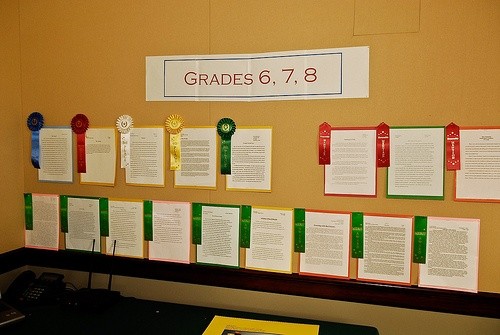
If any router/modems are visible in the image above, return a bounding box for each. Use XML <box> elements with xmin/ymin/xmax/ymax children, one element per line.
<box><xmin>60</xmin><ymin>239</ymin><xmax>123</xmax><ymax>315</ymax></box>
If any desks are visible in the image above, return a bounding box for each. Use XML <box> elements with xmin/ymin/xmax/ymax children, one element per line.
<box><xmin>1</xmin><ymin>299</ymin><xmax>378</xmax><ymax>335</ymax></box>
